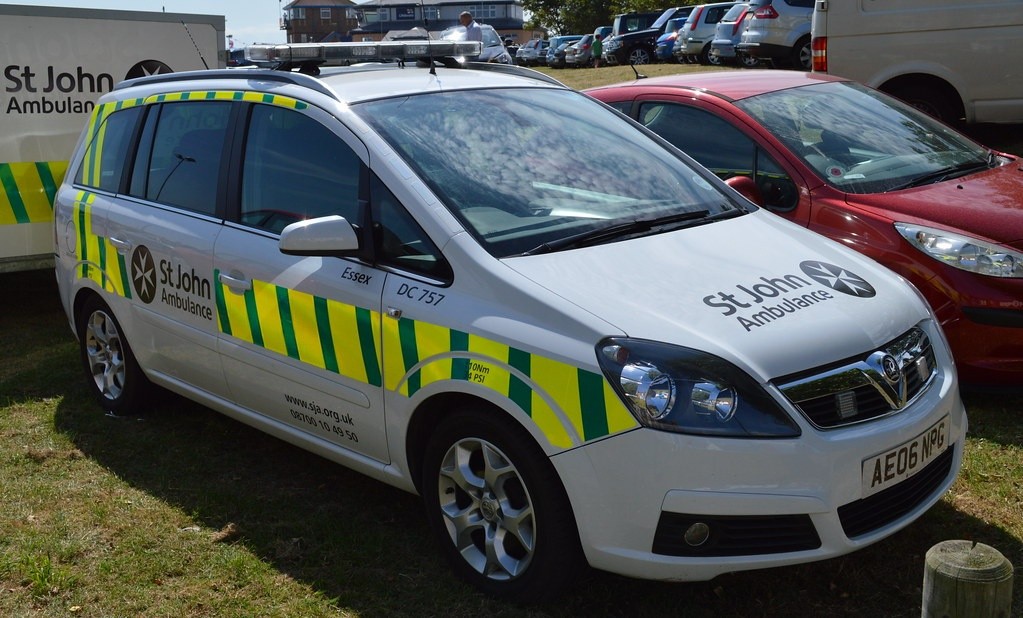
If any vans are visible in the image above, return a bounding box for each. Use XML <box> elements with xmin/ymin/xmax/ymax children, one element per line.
<box><xmin>811</xmin><ymin>0</ymin><xmax>1022</xmax><ymax>130</ymax></box>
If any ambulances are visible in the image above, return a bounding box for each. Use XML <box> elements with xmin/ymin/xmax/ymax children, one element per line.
<box><xmin>52</xmin><ymin>62</ymin><xmax>970</xmax><ymax>602</ymax></box>
<box><xmin>0</xmin><ymin>4</ymin><xmax>225</xmax><ymax>272</ymax></box>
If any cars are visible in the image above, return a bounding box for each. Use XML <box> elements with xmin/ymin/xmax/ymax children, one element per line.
<box><xmin>708</xmin><ymin>4</ymin><xmax>770</xmax><ymax>69</ymax></box>
<box><xmin>526</xmin><ymin>69</ymin><xmax>1023</xmax><ymax>388</ymax></box>
<box><xmin>656</xmin><ymin>18</ymin><xmax>699</xmax><ymax>64</ymax></box>
<box><xmin>439</xmin><ymin>23</ymin><xmax>513</xmax><ymax>65</ymax></box>
<box><xmin>680</xmin><ymin>2</ymin><xmax>749</xmax><ymax>66</ymax></box>
<box><xmin>563</xmin><ymin>9</ymin><xmax>686</xmax><ymax>66</ymax></box>
<box><xmin>515</xmin><ymin>35</ymin><xmax>583</xmax><ymax>69</ymax></box>
<box><xmin>605</xmin><ymin>4</ymin><xmax>728</xmax><ymax>65</ymax></box>
<box><xmin>734</xmin><ymin>0</ymin><xmax>815</xmax><ymax>70</ymax></box>
<box><xmin>240</xmin><ymin>209</ymin><xmax>314</xmax><ymax>235</ymax></box>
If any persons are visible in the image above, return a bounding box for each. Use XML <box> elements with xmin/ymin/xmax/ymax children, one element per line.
<box><xmin>459</xmin><ymin>11</ymin><xmax>482</xmax><ymax>61</ymax></box>
<box><xmin>589</xmin><ymin>35</ymin><xmax>604</xmax><ymax>68</ymax></box>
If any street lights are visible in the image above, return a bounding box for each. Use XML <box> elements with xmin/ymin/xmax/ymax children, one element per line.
<box><xmin>155</xmin><ymin>153</ymin><xmax>197</xmax><ymax>202</ymax></box>
